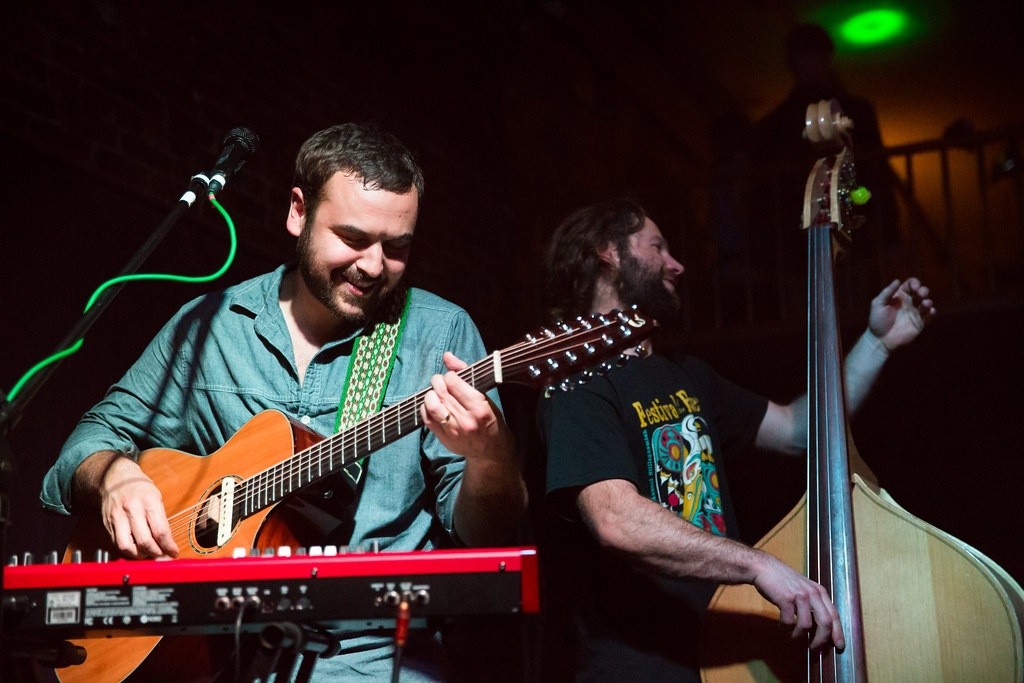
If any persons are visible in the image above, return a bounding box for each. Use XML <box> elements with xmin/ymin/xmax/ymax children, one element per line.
<box><xmin>527</xmin><ymin>196</ymin><xmax>935</xmax><ymax>683</ymax></box>
<box><xmin>39</xmin><ymin>124</ymin><xmax>529</xmax><ymax>682</ymax></box>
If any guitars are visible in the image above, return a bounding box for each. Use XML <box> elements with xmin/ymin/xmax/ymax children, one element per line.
<box><xmin>49</xmin><ymin>298</ymin><xmax>668</xmax><ymax>682</ymax></box>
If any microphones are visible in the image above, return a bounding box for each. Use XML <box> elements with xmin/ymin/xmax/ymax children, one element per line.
<box><xmin>207</xmin><ymin>124</ymin><xmax>261</xmax><ymax>194</ymax></box>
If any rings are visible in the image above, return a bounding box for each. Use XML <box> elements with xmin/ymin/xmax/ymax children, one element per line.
<box><xmin>440</xmin><ymin>414</ymin><xmax>450</xmax><ymax>426</ymax></box>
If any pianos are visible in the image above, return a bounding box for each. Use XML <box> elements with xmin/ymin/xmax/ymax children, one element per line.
<box><xmin>1</xmin><ymin>539</ymin><xmax>542</xmax><ymax>683</ymax></box>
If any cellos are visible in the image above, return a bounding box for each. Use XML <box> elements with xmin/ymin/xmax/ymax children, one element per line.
<box><xmin>696</xmin><ymin>94</ymin><xmax>1024</xmax><ymax>683</ymax></box>
